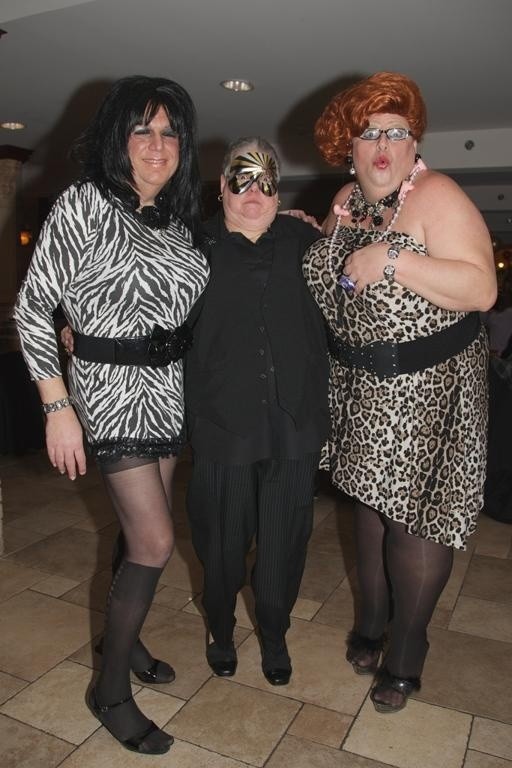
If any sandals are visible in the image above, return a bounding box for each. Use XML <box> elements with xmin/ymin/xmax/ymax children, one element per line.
<box><xmin>369</xmin><ymin>638</ymin><xmax>431</xmax><ymax>713</ymax></box>
<box><xmin>93</xmin><ymin>632</ymin><xmax>176</xmax><ymax>685</ymax></box>
<box><xmin>88</xmin><ymin>682</ymin><xmax>171</xmax><ymax>756</ymax></box>
<box><xmin>345</xmin><ymin>625</ymin><xmax>386</xmax><ymax>675</ymax></box>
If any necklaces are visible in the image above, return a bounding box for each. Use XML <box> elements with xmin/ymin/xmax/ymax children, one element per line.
<box><xmin>327</xmin><ymin>156</ymin><xmax>428</xmax><ymax>285</ymax></box>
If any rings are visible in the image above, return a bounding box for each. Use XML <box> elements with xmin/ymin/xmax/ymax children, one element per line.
<box><xmin>339</xmin><ymin>275</ymin><xmax>355</xmax><ymax>290</ymax></box>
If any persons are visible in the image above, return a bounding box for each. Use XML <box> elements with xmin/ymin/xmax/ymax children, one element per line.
<box><xmin>13</xmin><ymin>76</ymin><xmax>322</xmax><ymax>755</ymax></box>
<box><xmin>301</xmin><ymin>71</ymin><xmax>499</xmax><ymax>713</ymax></box>
<box><xmin>61</xmin><ymin>134</ymin><xmax>331</xmax><ymax>687</ymax></box>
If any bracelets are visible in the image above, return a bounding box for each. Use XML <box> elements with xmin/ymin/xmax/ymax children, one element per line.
<box><xmin>42</xmin><ymin>397</ymin><xmax>71</xmax><ymax>415</ymax></box>
<box><xmin>384</xmin><ymin>245</ymin><xmax>401</xmax><ymax>282</ymax></box>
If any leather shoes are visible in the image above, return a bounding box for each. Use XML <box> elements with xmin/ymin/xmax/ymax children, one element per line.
<box><xmin>259</xmin><ymin>629</ymin><xmax>293</xmax><ymax>686</ymax></box>
<box><xmin>205</xmin><ymin>626</ymin><xmax>238</xmax><ymax>678</ymax></box>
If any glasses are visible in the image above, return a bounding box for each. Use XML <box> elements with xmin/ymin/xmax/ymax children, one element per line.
<box><xmin>359</xmin><ymin>127</ymin><xmax>413</xmax><ymax>142</ymax></box>
